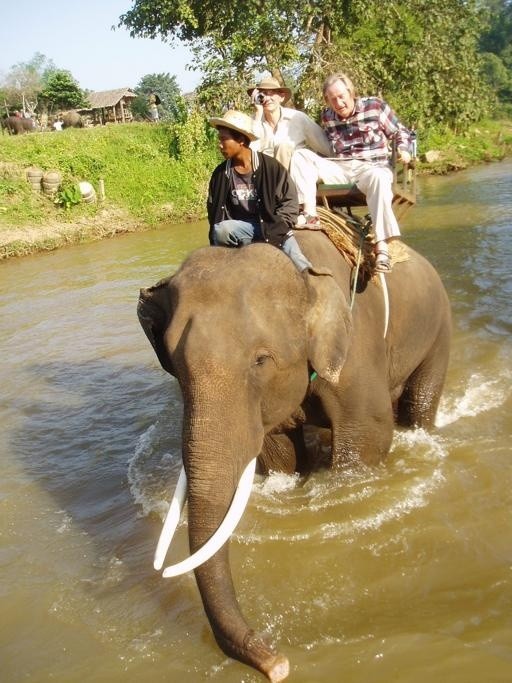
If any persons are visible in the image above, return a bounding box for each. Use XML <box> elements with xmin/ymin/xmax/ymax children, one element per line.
<box><xmin>206</xmin><ymin>75</ymin><xmax>333</xmax><ymax>275</ymax></box>
<box><xmin>146</xmin><ymin>89</ymin><xmax>159</xmax><ymax>122</ymax></box>
<box><xmin>53</xmin><ymin>119</ymin><xmax>64</xmax><ymax>131</ymax></box>
<box><xmin>289</xmin><ymin>72</ymin><xmax>410</xmax><ymax>273</ymax></box>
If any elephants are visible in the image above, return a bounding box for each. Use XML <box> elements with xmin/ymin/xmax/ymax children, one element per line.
<box><xmin>58</xmin><ymin>111</ymin><xmax>84</xmax><ymax>130</ymax></box>
<box><xmin>137</xmin><ymin>204</ymin><xmax>453</xmax><ymax>683</ymax></box>
<box><xmin>0</xmin><ymin>116</ymin><xmax>36</xmax><ymax>136</ymax></box>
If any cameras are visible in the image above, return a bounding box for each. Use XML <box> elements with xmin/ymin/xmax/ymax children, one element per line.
<box><xmin>253</xmin><ymin>92</ymin><xmax>268</xmax><ymax>106</ymax></box>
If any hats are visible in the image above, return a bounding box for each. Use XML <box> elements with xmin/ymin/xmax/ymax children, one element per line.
<box><xmin>209</xmin><ymin>110</ymin><xmax>262</xmax><ymax>142</ymax></box>
<box><xmin>245</xmin><ymin>76</ymin><xmax>292</xmax><ymax>105</ymax></box>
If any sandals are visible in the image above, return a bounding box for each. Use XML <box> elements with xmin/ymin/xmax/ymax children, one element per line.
<box><xmin>373</xmin><ymin>244</ymin><xmax>392</xmax><ymax>273</ymax></box>
<box><xmin>292</xmin><ymin>210</ymin><xmax>321</xmax><ymax>230</ymax></box>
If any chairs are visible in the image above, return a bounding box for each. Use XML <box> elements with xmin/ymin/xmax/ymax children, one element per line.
<box><xmin>318</xmin><ymin>130</ymin><xmax>416</xmax><ymax>227</ymax></box>
